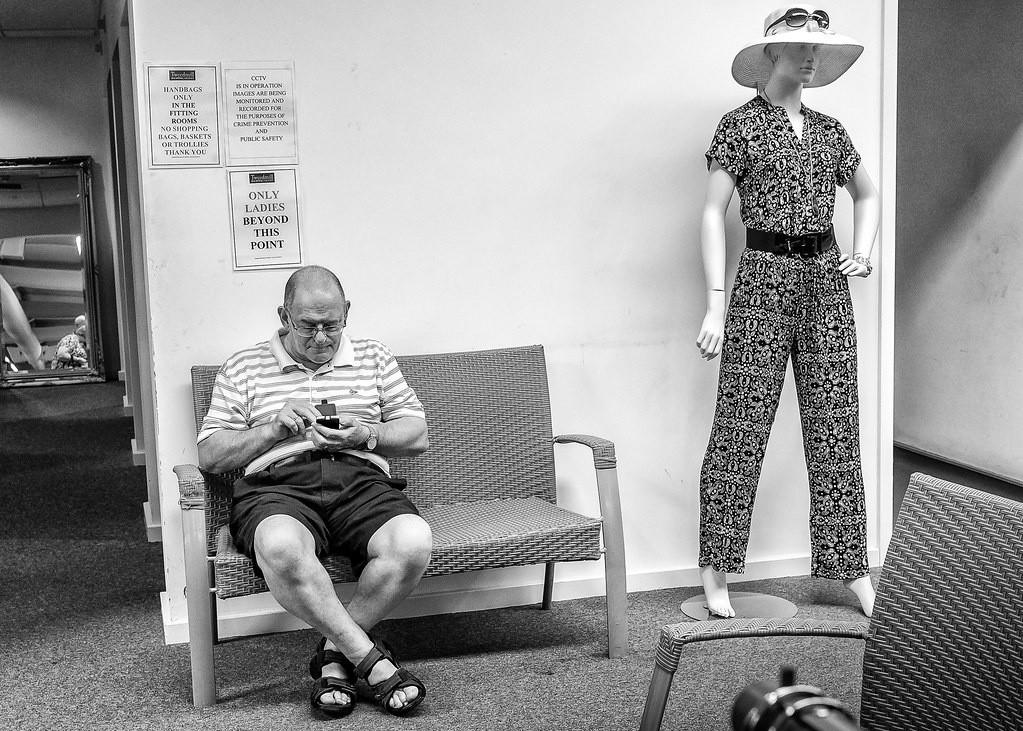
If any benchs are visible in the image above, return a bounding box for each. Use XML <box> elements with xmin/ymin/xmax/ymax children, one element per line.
<box><xmin>640</xmin><ymin>474</ymin><xmax>1023</xmax><ymax>731</ymax></box>
<box><xmin>171</xmin><ymin>343</ymin><xmax>628</xmax><ymax>706</ymax></box>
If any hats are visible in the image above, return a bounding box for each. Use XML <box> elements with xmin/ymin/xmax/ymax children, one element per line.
<box><xmin>74</xmin><ymin>315</ymin><xmax>86</xmax><ymax>333</ymax></box>
<box><xmin>732</xmin><ymin>4</ymin><xmax>863</xmax><ymax>88</ymax></box>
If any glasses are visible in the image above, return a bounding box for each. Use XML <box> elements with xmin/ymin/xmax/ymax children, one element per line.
<box><xmin>764</xmin><ymin>8</ymin><xmax>830</xmax><ymax>36</ymax></box>
<box><xmin>285</xmin><ymin>306</ymin><xmax>348</xmax><ymax>337</ymax></box>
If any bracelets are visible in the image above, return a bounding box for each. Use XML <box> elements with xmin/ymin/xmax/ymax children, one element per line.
<box><xmin>852</xmin><ymin>252</ymin><xmax>872</xmax><ymax>278</ymax></box>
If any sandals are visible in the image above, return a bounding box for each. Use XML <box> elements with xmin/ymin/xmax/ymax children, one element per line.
<box><xmin>353</xmin><ymin>630</ymin><xmax>426</xmax><ymax>716</ymax></box>
<box><xmin>311</xmin><ymin>635</ymin><xmax>357</xmax><ymax>715</ymax></box>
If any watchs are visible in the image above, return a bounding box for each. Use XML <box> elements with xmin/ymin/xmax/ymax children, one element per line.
<box><xmin>358</xmin><ymin>424</ymin><xmax>378</xmax><ymax>451</ymax></box>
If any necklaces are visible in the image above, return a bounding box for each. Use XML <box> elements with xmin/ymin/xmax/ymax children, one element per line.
<box><xmin>763</xmin><ymin>88</ymin><xmax>819</xmax><ymax>214</ymax></box>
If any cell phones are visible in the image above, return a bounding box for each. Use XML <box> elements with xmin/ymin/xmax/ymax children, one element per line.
<box><xmin>316</xmin><ymin>415</ymin><xmax>339</xmax><ymax>430</ymax></box>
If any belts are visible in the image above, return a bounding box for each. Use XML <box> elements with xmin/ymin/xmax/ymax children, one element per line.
<box><xmin>275</xmin><ymin>449</ymin><xmax>377</xmax><ymax>467</ymax></box>
<box><xmin>746</xmin><ymin>224</ymin><xmax>836</xmax><ymax>259</ymax></box>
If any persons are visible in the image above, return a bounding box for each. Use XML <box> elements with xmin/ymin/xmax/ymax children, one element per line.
<box><xmin>0</xmin><ymin>274</ymin><xmax>89</xmax><ymax>371</ymax></box>
<box><xmin>698</xmin><ymin>7</ymin><xmax>878</xmax><ymax>617</ymax></box>
<box><xmin>198</xmin><ymin>266</ymin><xmax>433</xmax><ymax>716</ymax></box>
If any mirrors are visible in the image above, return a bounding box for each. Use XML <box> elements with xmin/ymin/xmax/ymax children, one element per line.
<box><xmin>0</xmin><ymin>156</ymin><xmax>109</xmax><ymax>388</ymax></box>
